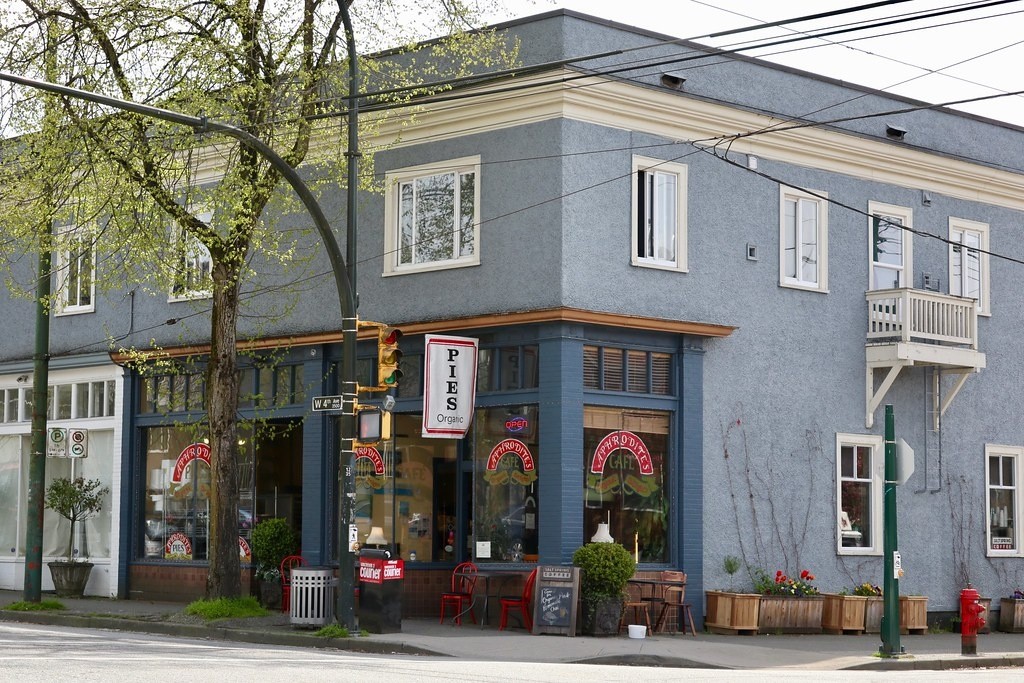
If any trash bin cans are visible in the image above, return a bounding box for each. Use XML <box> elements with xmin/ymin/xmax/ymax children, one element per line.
<box><xmin>357</xmin><ymin>546</ymin><xmax>406</xmax><ymax>634</ymax></box>
<box><xmin>287</xmin><ymin>565</ymin><xmax>341</xmax><ymax>629</ymax></box>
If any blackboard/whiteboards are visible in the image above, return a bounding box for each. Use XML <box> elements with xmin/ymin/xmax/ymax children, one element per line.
<box><xmin>533</xmin><ymin>584</ymin><xmax>574</xmax><ymax>627</ymax></box>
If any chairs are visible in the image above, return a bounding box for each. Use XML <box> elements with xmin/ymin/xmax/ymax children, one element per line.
<box><xmin>439</xmin><ymin>563</ymin><xmax>476</xmax><ymax>625</ymax></box>
<box><xmin>499</xmin><ymin>566</ymin><xmax>538</xmax><ymax>631</ymax></box>
<box><xmin>281</xmin><ymin>556</ymin><xmax>308</xmax><ymax>615</ymax></box>
<box><xmin>617</xmin><ymin>587</ymin><xmax>652</xmax><ymax>636</ymax></box>
<box><xmin>654</xmin><ymin>571</ymin><xmax>697</xmax><ymax>636</ymax></box>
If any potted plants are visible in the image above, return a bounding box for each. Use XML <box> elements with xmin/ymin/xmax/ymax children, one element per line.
<box><xmin>249</xmin><ymin>517</ymin><xmax>297</xmax><ymax>609</ymax></box>
<box><xmin>820</xmin><ymin>586</ymin><xmax>869</xmax><ymax>637</ymax></box>
<box><xmin>43</xmin><ymin>472</ymin><xmax>111</xmax><ymax>599</ymax></box>
<box><xmin>959</xmin><ymin>588</ymin><xmax>992</xmax><ymax>634</ymax></box>
<box><xmin>571</xmin><ymin>541</ymin><xmax>637</xmax><ymax>637</ymax></box>
<box><xmin>899</xmin><ymin>594</ymin><xmax>928</xmax><ymax>636</ymax></box>
<box><xmin>704</xmin><ymin>555</ymin><xmax>762</xmax><ymax>635</ymax></box>
<box><xmin>950</xmin><ymin>615</ymin><xmax>962</xmax><ymax>633</ymax></box>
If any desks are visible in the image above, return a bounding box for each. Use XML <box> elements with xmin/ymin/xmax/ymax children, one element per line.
<box><xmin>626</xmin><ymin>580</ymin><xmax>686</xmax><ymax>636</ymax></box>
<box><xmin>451</xmin><ymin>572</ymin><xmax>515</xmax><ymax>632</ymax></box>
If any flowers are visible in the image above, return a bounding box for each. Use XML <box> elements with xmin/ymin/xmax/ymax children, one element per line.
<box><xmin>853</xmin><ymin>583</ymin><xmax>883</xmax><ymax>596</ymax></box>
<box><xmin>753</xmin><ymin>566</ymin><xmax>820</xmax><ymax>596</ymax></box>
<box><xmin>1010</xmin><ymin>589</ymin><xmax>1024</xmax><ymax>599</ymax></box>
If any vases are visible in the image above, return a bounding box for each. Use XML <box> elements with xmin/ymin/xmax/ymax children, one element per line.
<box><xmin>758</xmin><ymin>597</ymin><xmax>822</xmax><ymax>635</ymax></box>
<box><xmin>998</xmin><ymin>598</ymin><xmax>1024</xmax><ymax>633</ymax></box>
<box><xmin>865</xmin><ymin>596</ymin><xmax>887</xmax><ymax>633</ymax></box>
